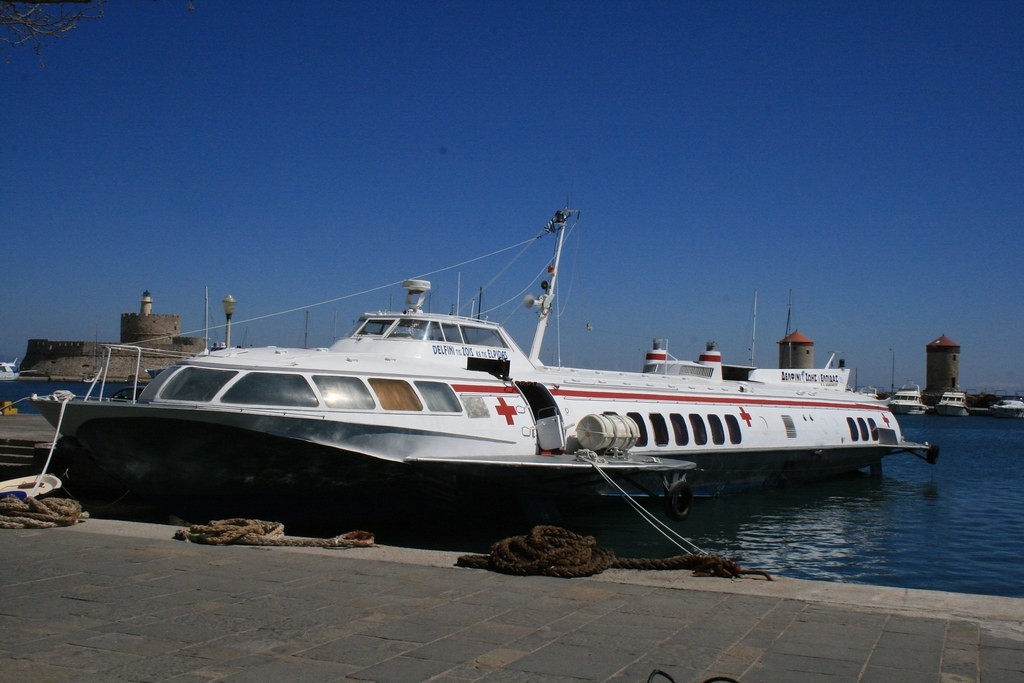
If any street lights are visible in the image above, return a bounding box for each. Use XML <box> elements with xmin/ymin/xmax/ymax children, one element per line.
<box><xmin>888</xmin><ymin>347</ymin><xmax>895</xmax><ymax>396</ymax></box>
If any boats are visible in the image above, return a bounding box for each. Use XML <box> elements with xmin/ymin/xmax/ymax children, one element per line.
<box><xmin>934</xmin><ymin>390</ymin><xmax>971</xmax><ymax>417</ymax></box>
<box><xmin>886</xmin><ymin>379</ymin><xmax>929</xmax><ymax>415</ymax></box>
<box><xmin>29</xmin><ymin>195</ymin><xmax>940</xmax><ymax>523</ymax></box>
<box><xmin>986</xmin><ymin>399</ymin><xmax>1024</xmax><ymax>418</ymax></box>
